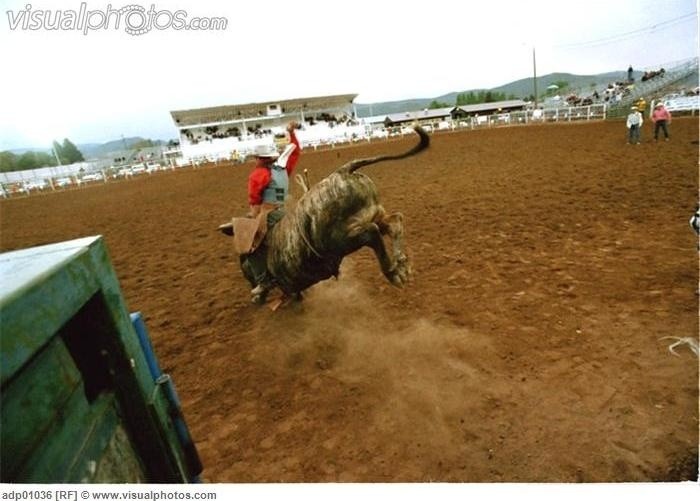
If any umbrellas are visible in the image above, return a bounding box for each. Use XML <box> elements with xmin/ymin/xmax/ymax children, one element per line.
<box><xmin>547</xmin><ymin>85</ymin><xmax>560</xmax><ymax>97</ymax></box>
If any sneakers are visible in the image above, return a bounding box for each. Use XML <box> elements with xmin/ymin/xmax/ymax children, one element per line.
<box><xmin>251</xmin><ymin>285</ymin><xmax>265</xmax><ymax>294</ymax></box>
<box><xmin>626</xmin><ymin>138</ymin><xmax>669</xmax><ymax>144</ymax></box>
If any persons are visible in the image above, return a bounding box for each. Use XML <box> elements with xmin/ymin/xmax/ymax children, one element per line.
<box><xmin>181</xmin><ymin>110</ymin><xmax>351</xmax><ymax>142</ymax></box>
<box><xmin>245</xmin><ymin>119</ymin><xmax>305</xmax><ymax>299</ymax></box>
<box><xmin>624</xmin><ymin>106</ymin><xmax>646</xmax><ymax>146</ymax></box>
<box><xmin>626</xmin><ymin>65</ymin><xmax>634</xmax><ymax>82</ymax></box>
<box><xmin>651</xmin><ymin>103</ymin><xmax>672</xmax><ymax>142</ymax></box>
<box><xmin>635</xmin><ymin>97</ymin><xmax>647</xmax><ymax>119</ymax></box>
<box><xmin>571</xmin><ymin>68</ymin><xmax>667</xmax><ymax>107</ymax></box>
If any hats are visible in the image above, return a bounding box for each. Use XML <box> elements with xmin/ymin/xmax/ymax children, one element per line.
<box><xmin>253</xmin><ymin>144</ymin><xmax>280</xmax><ymax>157</ymax></box>
<box><xmin>656</xmin><ymin>103</ymin><xmax>664</xmax><ymax>107</ymax></box>
<box><xmin>631</xmin><ymin>106</ymin><xmax>638</xmax><ymax>109</ymax></box>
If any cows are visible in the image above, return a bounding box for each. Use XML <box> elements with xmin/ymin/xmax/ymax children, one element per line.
<box><xmin>213</xmin><ymin>123</ymin><xmax>431</xmax><ymax>306</ymax></box>
